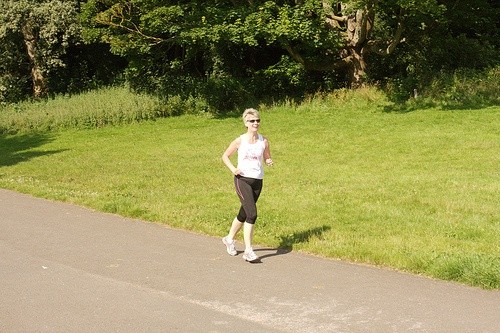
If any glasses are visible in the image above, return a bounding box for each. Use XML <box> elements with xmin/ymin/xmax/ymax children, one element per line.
<box><xmin>247</xmin><ymin>119</ymin><xmax>260</xmax><ymax>123</ymax></box>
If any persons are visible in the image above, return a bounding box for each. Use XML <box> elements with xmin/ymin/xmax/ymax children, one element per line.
<box><xmin>222</xmin><ymin>108</ymin><xmax>273</xmax><ymax>263</ymax></box>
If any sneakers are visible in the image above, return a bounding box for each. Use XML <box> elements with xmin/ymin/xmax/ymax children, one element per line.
<box><xmin>242</xmin><ymin>249</ymin><xmax>261</xmax><ymax>263</ymax></box>
<box><xmin>222</xmin><ymin>236</ymin><xmax>237</xmax><ymax>256</ymax></box>
